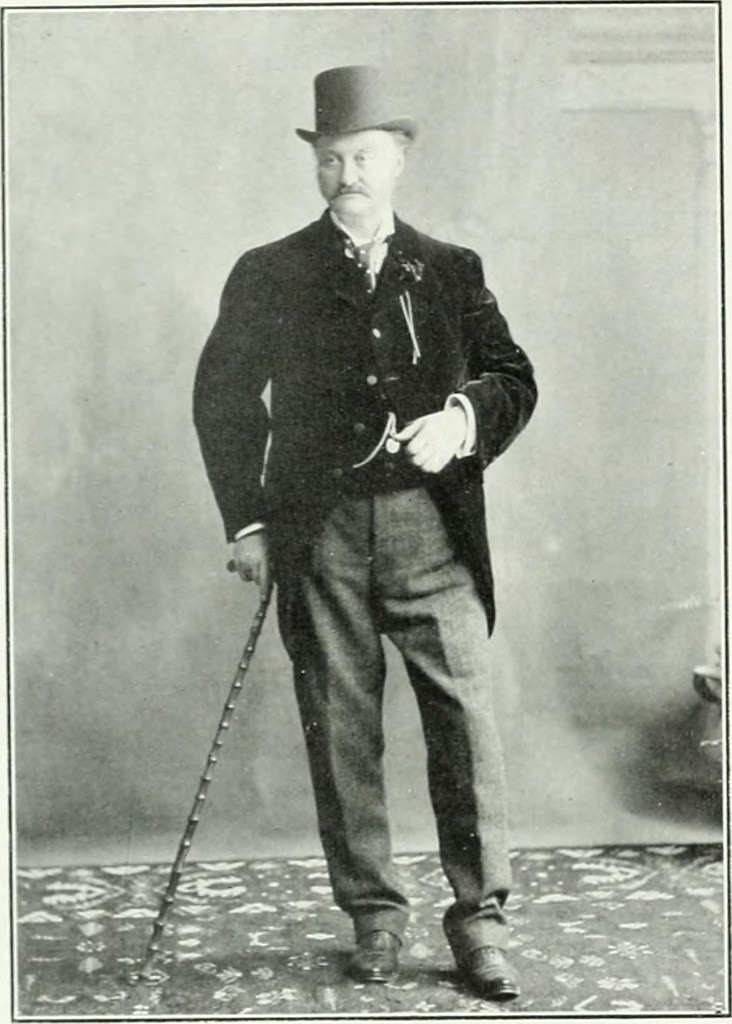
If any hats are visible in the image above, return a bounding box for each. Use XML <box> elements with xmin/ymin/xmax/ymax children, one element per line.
<box><xmin>295</xmin><ymin>64</ymin><xmax>416</xmax><ymax>145</ymax></box>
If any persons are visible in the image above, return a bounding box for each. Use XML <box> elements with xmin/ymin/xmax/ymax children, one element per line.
<box><xmin>189</xmin><ymin>66</ymin><xmax>542</xmax><ymax>1000</ymax></box>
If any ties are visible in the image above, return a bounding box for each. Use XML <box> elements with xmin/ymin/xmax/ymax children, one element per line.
<box><xmin>338</xmin><ymin>229</ymin><xmax>387</xmax><ymax>293</ymax></box>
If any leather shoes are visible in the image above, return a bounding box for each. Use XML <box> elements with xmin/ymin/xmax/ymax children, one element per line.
<box><xmin>460</xmin><ymin>947</ymin><xmax>521</xmax><ymax>1000</ymax></box>
<box><xmin>349</xmin><ymin>931</ymin><xmax>403</xmax><ymax>983</ymax></box>
